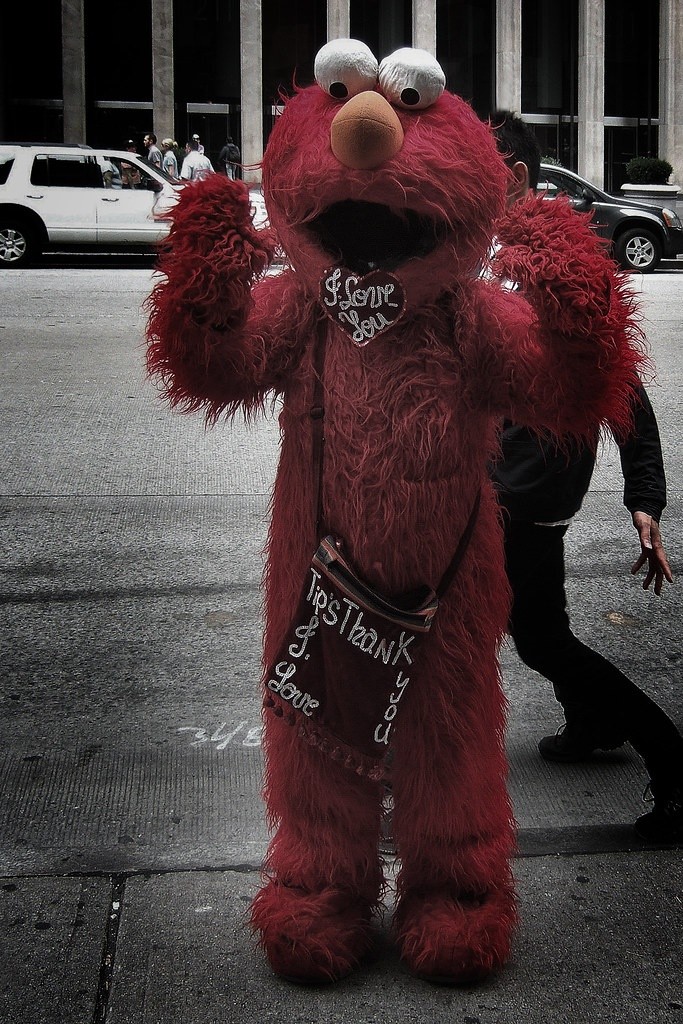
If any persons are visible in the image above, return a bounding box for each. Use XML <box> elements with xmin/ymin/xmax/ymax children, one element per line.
<box><xmin>144</xmin><ymin>133</ymin><xmax>163</xmax><ymax>191</ymax></box>
<box><xmin>219</xmin><ymin>136</ymin><xmax>240</xmax><ymax>182</ymax></box>
<box><xmin>192</xmin><ymin>134</ymin><xmax>204</xmax><ymax>155</ymax></box>
<box><xmin>121</xmin><ymin>138</ymin><xmax>141</xmax><ymax>191</ymax></box>
<box><xmin>472</xmin><ymin>108</ymin><xmax>683</xmax><ymax>845</ymax></box>
<box><xmin>180</xmin><ymin>141</ymin><xmax>216</xmax><ymax>183</ymax></box>
<box><xmin>161</xmin><ymin>138</ymin><xmax>178</xmax><ymax>180</ymax></box>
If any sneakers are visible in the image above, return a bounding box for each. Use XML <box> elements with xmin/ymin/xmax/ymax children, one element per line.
<box><xmin>634</xmin><ymin>781</ymin><xmax>683</xmax><ymax>840</ymax></box>
<box><xmin>537</xmin><ymin>719</ymin><xmax>609</xmax><ymax>763</ymax></box>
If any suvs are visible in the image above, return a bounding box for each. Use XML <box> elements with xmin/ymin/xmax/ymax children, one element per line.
<box><xmin>0</xmin><ymin>142</ymin><xmax>185</xmax><ymax>269</ymax></box>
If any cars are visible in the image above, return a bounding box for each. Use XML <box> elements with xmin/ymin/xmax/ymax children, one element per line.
<box><xmin>537</xmin><ymin>163</ymin><xmax>683</xmax><ymax>275</ymax></box>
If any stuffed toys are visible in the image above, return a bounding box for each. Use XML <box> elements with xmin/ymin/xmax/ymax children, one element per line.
<box><xmin>139</xmin><ymin>37</ymin><xmax>651</xmax><ymax>987</ymax></box>
